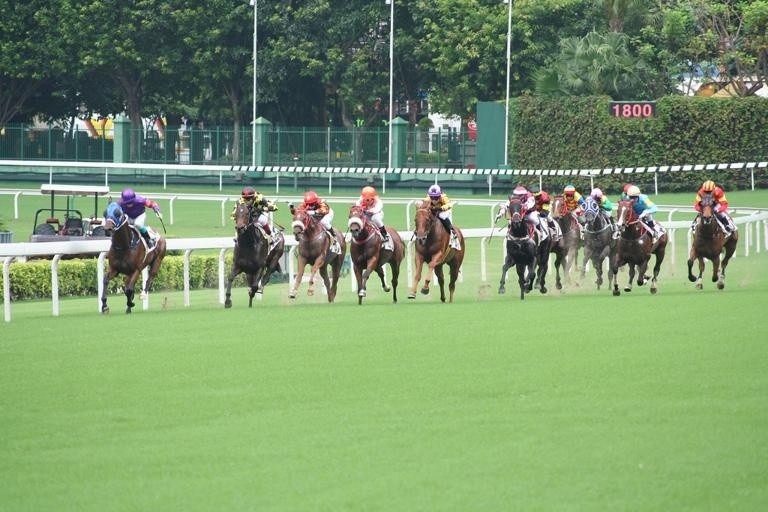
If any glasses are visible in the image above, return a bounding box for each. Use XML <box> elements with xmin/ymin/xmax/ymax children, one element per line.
<box><xmin>430</xmin><ymin>197</ymin><xmax>439</xmax><ymax>201</ymax></box>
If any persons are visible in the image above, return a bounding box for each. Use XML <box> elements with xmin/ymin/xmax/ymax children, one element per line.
<box><xmin>114</xmin><ymin>187</ymin><xmax>161</xmax><ymax>250</ymax></box>
<box><xmin>287</xmin><ymin>190</ymin><xmax>336</xmax><ymax>243</ymax></box>
<box><xmin>505</xmin><ymin>183</ymin><xmax>664</xmax><ymax>246</ymax></box>
<box><xmin>691</xmin><ymin>179</ymin><xmax>737</xmax><ymax>240</ymax></box>
<box><xmin>352</xmin><ymin>185</ymin><xmax>389</xmax><ymax>241</ymax></box>
<box><xmin>228</xmin><ymin>185</ymin><xmax>280</xmax><ymax>246</ymax></box>
<box><xmin>421</xmin><ymin>184</ymin><xmax>460</xmax><ymax>244</ymax></box>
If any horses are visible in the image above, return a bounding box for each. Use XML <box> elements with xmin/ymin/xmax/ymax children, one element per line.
<box><xmin>348</xmin><ymin>200</ymin><xmax>406</xmax><ymax>305</ymax></box>
<box><xmin>407</xmin><ymin>198</ymin><xmax>465</xmax><ymax>304</ymax></box>
<box><xmin>100</xmin><ymin>195</ymin><xmax>167</xmax><ymax>315</ymax></box>
<box><xmin>224</xmin><ymin>199</ymin><xmax>285</xmax><ymax>308</ymax></box>
<box><xmin>687</xmin><ymin>190</ymin><xmax>739</xmax><ymax>290</ymax></box>
<box><xmin>497</xmin><ymin>192</ymin><xmax>668</xmax><ymax>300</ymax></box>
<box><xmin>288</xmin><ymin>203</ymin><xmax>346</xmax><ymax>304</ymax></box>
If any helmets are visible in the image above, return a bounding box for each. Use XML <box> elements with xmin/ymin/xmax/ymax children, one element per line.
<box><xmin>623</xmin><ymin>183</ymin><xmax>632</xmax><ymax>193</ymax></box>
<box><xmin>361</xmin><ymin>186</ymin><xmax>376</xmax><ymax>199</ymax></box>
<box><xmin>703</xmin><ymin>180</ymin><xmax>715</xmax><ymax>192</ymax></box>
<box><xmin>591</xmin><ymin>188</ymin><xmax>603</xmax><ymax>199</ymax></box>
<box><xmin>242</xmin><ymin>187</ymin><xmax>257</xmax><ymax>198</ymax></box>
<box><xmin>120</xmin><ymin>188</ymin><xmax>137</xmax><ymax>205</ymax></box>
<box><xmin>513</xmin><ymin>186</ymin><xmax>528</xmax><ymax>195</ymax></box>
<box><xmin>428</xmin><ymin>184</ymin><xmax>442</xmax><ymax>197</ymax></box>
<box><xmin>564</xmin><ymin>185</ymin><xmax>576</xmax><ymax>196</ymax></box>
<box><xmin>627</xmin><ymin>186</ymin><xmax>640</xmax><ymax>197</ymax></box>
<box><xmin>529</xmin><ymin>188</ymin><xmax>542</xmax><ymax>200</ymax></box>
<box><xmin>303</xmin><ymin>191</ymin><xmax>318</xmax><ymax>204</ymax></box>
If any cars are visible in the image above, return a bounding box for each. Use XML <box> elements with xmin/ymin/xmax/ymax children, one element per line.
<box><xmin>666</xmin><ymin>56</ymin><xmax>768</xmax><ymax>100</ymax></box>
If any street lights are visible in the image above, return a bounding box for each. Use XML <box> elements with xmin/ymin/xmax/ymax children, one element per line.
<box><xmin>502</xmin><ymin>0</ymin><xmax>513</xmax><ymax>169</ymax></box>
<box><xmin>249</xmin><ymin>0</ymin><xmax>262</xmax><ymax>168</ymax></box>
<box><xmin>384</xmin><ymin>0</ymin><xmax>396</xmax><ymax>168</ymax></box>
